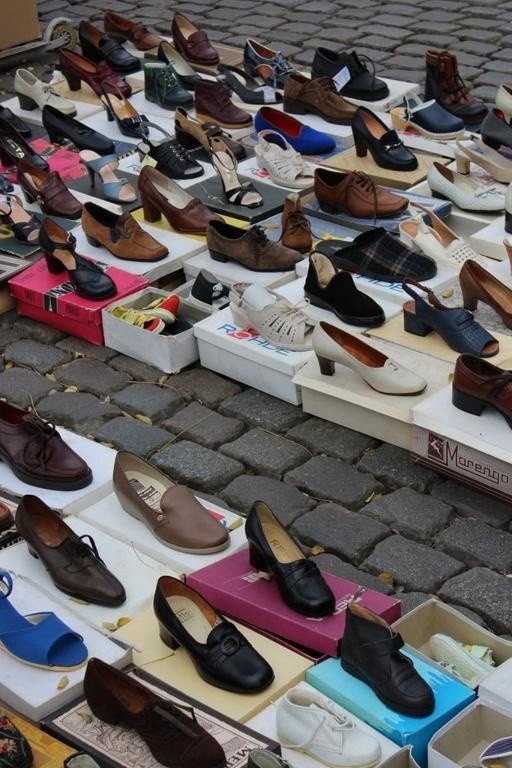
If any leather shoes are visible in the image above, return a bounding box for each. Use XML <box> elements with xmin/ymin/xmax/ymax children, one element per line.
<box><xmin>112</xmin><ymin>450</ymin><xmax>230</xmax><ymax>554</ymax></box>
<box><xmin>341</xmin><ymin>604</ymin><xmax>435</xmax><ymax>716</ymax></box>
<box><xmin>84</xmin><ymin>657</ymin><xmax>228</xmax><ymax>767</ymax></box>
<box><xmin>1</xmin><ymin>401</ymin><xmax>92</xmax><ymax>490</ymax></box>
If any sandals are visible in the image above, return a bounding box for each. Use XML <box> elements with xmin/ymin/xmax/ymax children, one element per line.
<box><xmin>0</xmin><ymin>571</ymin><xmax>88</xmax><ymax>671</ymax></box>
<box><xmin>424</xmin><ymin>634</ymin><xmax>497</xmax><ymax>689</ymax></box>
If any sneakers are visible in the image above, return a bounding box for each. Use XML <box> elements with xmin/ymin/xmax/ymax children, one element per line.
<box><xmin>278</xmin><ymin>690</ymin><xmax>382</xmax><ymax>767</ymax></box>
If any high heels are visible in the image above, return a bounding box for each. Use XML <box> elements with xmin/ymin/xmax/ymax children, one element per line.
<box><xmin>154</xmin><ymin>576</ymin><xmax>275</xmax><ymax>694</ymax></box>
<box><xmin>0</xmin><ymin>11</ymin><xmax>228</xmax><ymax>336</ymax></box>
<box><xmin>245</xmin><ymin>503</ymin><xmax>335</xmax><ymax>617</ymax></box>
<box><xmin>229</xmin><ymin>47</ymin><xmax>512</xmax><ymax>428</ymax></box>
<box><xmin>16</xmin><ymin>495</ymin><xmax>126</xmax><ymax>607</ymax></box>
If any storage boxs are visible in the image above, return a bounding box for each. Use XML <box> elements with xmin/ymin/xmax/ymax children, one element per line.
<box><xmin>0</xmin><ymin>27</ymin><xmax>512</xmax><ymax>768</ymax></box>
